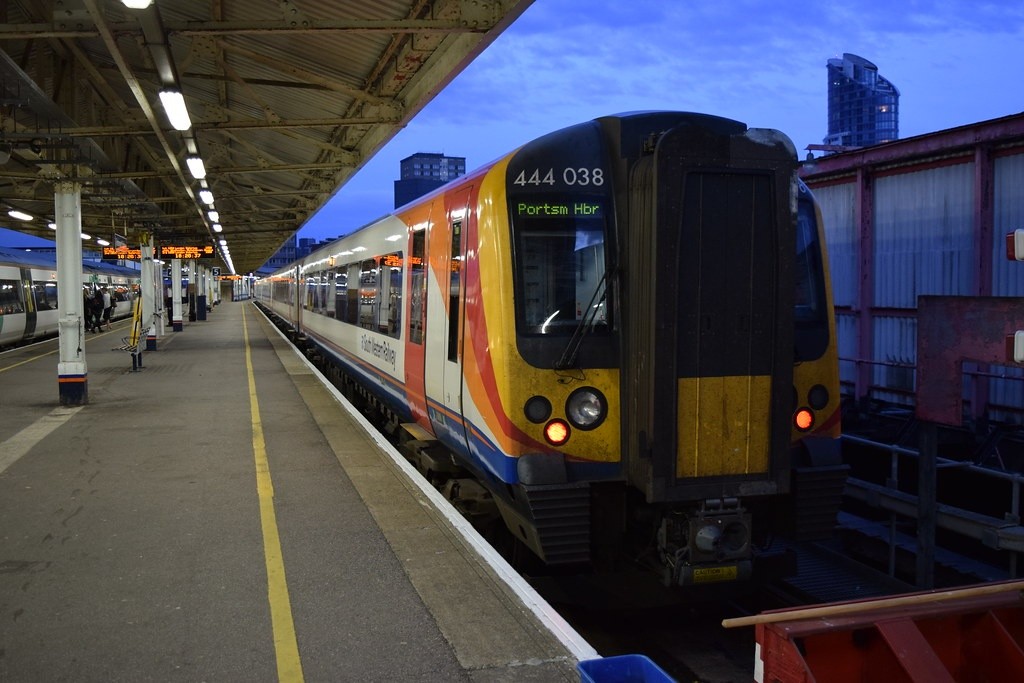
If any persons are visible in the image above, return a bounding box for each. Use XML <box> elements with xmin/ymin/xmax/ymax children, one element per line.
<box><xmin>83</xmin><ymin>287</ymin><xmax>117</xmax><ymax>334</ymax></box>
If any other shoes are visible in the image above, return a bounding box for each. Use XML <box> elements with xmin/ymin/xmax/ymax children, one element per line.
<box><xmin>84</xmin><ymin>330</ymin><xmax>90</xmax><ymax>333</ymax></box>
<box><xmin>89</xmin><ymin>325</ymin><xmax>92</xmax><ymax>328</ymax></box>
<box><xmin>107</xmin><ymin>327</ymin><xmax>110</xmax><ymax>332</ymax></box>
<box><xmin>91</xmin><ymin>327</ymin><xmax>96</xmax><ymax>334</ymax></box>
<box><xmin>106</xmin><ymin>326</ymin><xmax>112</xmax><ymax>328</ymax></box>
<box><xmin>99</xmin><ymin>328</ymin><xmax>104</xmax><ymax>332</ymax></box>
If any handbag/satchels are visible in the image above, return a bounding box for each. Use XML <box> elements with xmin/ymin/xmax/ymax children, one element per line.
<box><xmin>110</xmin><ymin>296</ymin><xmax>117</xmax><ymax>308</ymax></box>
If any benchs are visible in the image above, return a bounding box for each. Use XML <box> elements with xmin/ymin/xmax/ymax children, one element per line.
<box><xmin>111</xmin><ymin>325</ymin><xmax>152</xmax><ymax>372</ymax></box>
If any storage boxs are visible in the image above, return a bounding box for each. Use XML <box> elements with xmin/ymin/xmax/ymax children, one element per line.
<box><xmin>576</xmin><ymin>653</ymin><xmax>679</xmax><ymax>683</ymax></box>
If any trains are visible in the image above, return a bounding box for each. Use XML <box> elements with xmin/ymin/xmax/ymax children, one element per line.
<box><xmin>0</xmin><ymin>224</ymin><xmax>189</xmax><ymax>352</ymax></box>
<box><xmin>253</xmin><ymin>107</ymin><xmax>852</xmax><ymax>585</ymax></box>
<box><xmin>793</xmin><ymin>111</ymin><xmax>1024</xmax><ymax>518</ymax></box>
